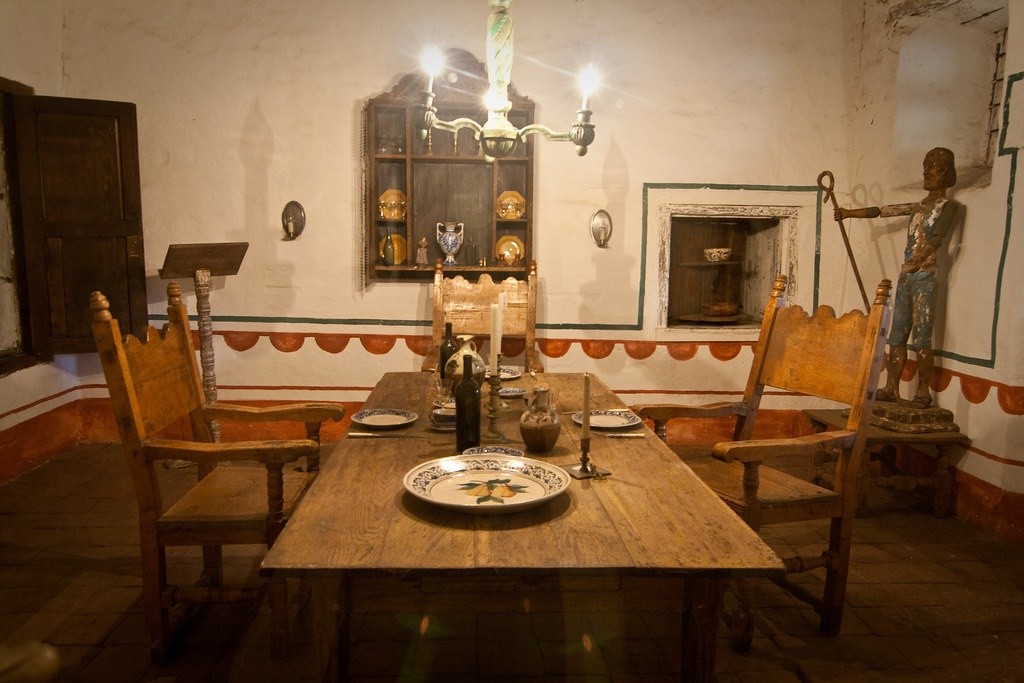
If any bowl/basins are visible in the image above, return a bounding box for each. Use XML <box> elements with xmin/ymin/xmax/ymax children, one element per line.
<box><xmin>702</xmin><ymin>303</ymin><xmax>739</xmax><ymax>316</ymax></box>
<box><xmin>703</xmin><ymin>248</ymin><xmax>731</xmax><ymax>261</ymax></box>
<box><xmin>432</xmin><ymin>409</ymin><xmax>456</xmax><ymax>427</ymax></box>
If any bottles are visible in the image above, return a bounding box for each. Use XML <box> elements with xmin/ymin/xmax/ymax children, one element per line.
<box><xmin>520</xmin><ymin>388</ymin><xmax>561</xmax><ymax>453</ymax></box>
<box><xmin>383</xmin><ymin>227</ymin><xmax>394</xmax><ymax>266</ymax></box>
<box><xmin>440</xmin><ymin>323</ymin><xmax>458</xmax><ymax>380</ymax></box>
<box><xmin>455</xmin><ymin>355</ymin><xmax>481</xmax><ymax>452</ymax></box>
<box><xmin>445</xmin><ymin>334</ymin><xmax>485</xmax><ymax>375</ymax></box>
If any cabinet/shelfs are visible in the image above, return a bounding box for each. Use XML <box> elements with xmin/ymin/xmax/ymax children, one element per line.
<box><xmin>364</xmin><ymin>47</ymin><xmax>537</xmax><ymax>282</ymax></box>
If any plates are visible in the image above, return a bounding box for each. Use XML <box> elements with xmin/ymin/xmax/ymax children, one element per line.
<box><xmin>495</xmin><ymin>235</ymin><xmax>524</xmax><ymax>265</ymax></box>
<box><xmin>350</xmin><ymin>407</ymin><xmax>418</xmax><ymax>428</ymax></box>
<box><xmin>429</xmin><ymin>425</ymin><xmax>456</xmax><ymax>431</ymax></box>
<box><xmin>485</xmin><ymin>368</ymin><xmax>521</xmax><ymax>380</ymax></box>
<box><xmin>377</xmin><ymin>189</ymin><xmax>406</xmax><ymax>220</ymax></box>
<box><xmin>379</xmin><ymin>234</ymin><xmax>407</xmax><ymax>265</ymax></box>
<box><xmin>497</xmin><ymin>387</ymin><xmax>526</xmax><ymax>398</ymax></box>
<box><xmin>402</xmin><ymin>452</ymin><xmax>572</xmax><ymax>516</ymax></box>
<box><xmin>496</xmin><ymin>190</ymin><xmax>526</xmax><ymax>220</ymax></box>
<box><xmin>571</xmin><ymin>408</ymin><xmax>641</xmax><ymax>428</ymax></box>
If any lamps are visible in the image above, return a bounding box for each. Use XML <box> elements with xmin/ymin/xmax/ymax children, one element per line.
<box><xmin>420</xmin><ymin>1</ymin><xmax>606</xmax><ymax>160</ymax></box>
<box><xmin>280</xmin><ymin>201</ymin><xmax>304</xmax><ymax>240</ymax></box>
<box><xmin>590</xmin><ymin>210</ymin><xmax>615</xmax><ymax>248</ymax></box>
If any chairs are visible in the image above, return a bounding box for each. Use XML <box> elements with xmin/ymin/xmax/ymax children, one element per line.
<box><xmin>636</xmin><ymin>279</ymin><xmax>896</xmax><ymax>653</ymax></box>
<box><xmin>87</xmin><ymin>281</ymin><xmax>345</xmax><ymax>653</ymax></box>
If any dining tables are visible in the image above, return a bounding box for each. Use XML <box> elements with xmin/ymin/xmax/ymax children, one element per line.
<box><xmin>262</xmin><ymin>371</ymin><xmax>785</xmax><ymax>682</ymax></box>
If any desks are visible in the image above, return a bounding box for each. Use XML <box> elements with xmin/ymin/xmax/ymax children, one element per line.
<box><xmin>802</xmin><ymin>408</ymin><xmax>970</xmax><ymax>519</ymax></box>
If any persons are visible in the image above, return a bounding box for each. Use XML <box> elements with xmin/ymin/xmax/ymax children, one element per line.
<box><xmin>833</xmin><ymin>146</ymin><xmax>967</xmax><ymax>410</ymax></box>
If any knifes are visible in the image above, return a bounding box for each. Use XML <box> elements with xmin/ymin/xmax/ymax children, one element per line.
<box><xmin>347</xmin><ymin>432</ymin><xmax>431</xmax><ymax>438</ymax></box>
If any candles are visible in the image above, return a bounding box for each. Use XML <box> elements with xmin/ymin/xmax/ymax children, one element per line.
<box><xmin>491</xmin><ymin>303</ymin><xmax>499</xmax><ymax>374</ymax></box>
<box><xmin>583</xmin><ymin>372</ymin><xmax>590</xmax><ymax>438</ymax></box>
<box><xmin>498</xmin><ymin>293</ymin><xmax>506</xmax><ymax>353</ymax></box>
